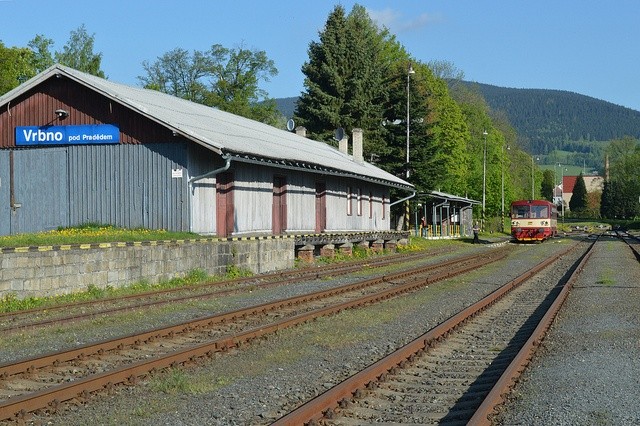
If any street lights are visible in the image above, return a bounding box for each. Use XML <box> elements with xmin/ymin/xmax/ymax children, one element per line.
<box><xmin>501</xmin><ymin>144</ymin><xmax>510</xmax><ymax>232</ymax></box>
<box><xmin>405</xmin><ymin>64</ymin><xmax>416</xmax><ymax>230</ymax></box>
<box><xmin>483</xmin><ymin>130</ymin><xmax>488</xmax><ymax>232</ymax></box>
<box><xmin>554</xmin><ymin>162</ymin><xmax>560</xmax><ymax>203</ymax></box>
<box><xmin>562</xmin><ymin>167</ymin><xmax>567</xmax><ymax>230</ymax></box>
<box><xmin>532</xmin><ymin>156</ymin><xmax>539</xmax><ymax>200</ymax></box>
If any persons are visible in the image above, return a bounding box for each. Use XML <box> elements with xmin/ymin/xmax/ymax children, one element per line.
<box><xmin>420</xmin><ymin>216</ymin><xmax>428</xmax><ymax>238</ymax></box>
<box><xmin>472</xmin><ymin>221</ymin><xmax>480</xmax><ymax>244</ymax></box>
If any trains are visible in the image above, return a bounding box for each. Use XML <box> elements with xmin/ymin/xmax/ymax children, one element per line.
<box><xmin>511</xmin><ymin>199</ymin><xmax>557</xmax><ymax>243</ymax></box>
